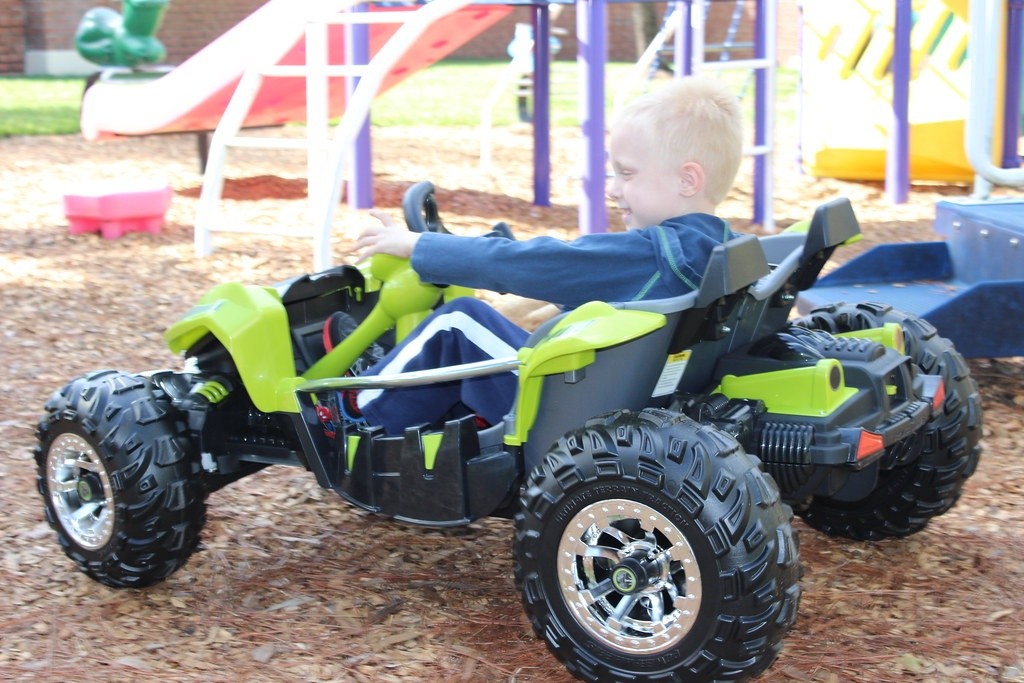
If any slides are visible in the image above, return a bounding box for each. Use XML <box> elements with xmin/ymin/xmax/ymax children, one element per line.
<box><xmin>80</xmin><ymin>0</ymin><xmax>509</xmax><ymax>138</ymax></box>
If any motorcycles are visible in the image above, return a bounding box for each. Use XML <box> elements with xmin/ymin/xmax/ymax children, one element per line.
<box><xmin>36</xmin><ymin>172</ymin><xmax>985</xmax><ymax>682</ymax></box>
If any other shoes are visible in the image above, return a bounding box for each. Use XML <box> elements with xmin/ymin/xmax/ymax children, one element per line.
<box><xmin>323</xmin><ymin>371</ymin><xmax>362</xmax><ymax>439</ymax></box>
<box><xmin>322</xmin><ymin>311</ymin><xmax>394</xmax><ymax>377</ymax></box>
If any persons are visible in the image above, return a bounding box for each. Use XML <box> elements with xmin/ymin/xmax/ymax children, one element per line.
<box><xmin>298</xmin><ymin>76</ymin><xmax>742</xmax><ymax>431</ymax></box>
